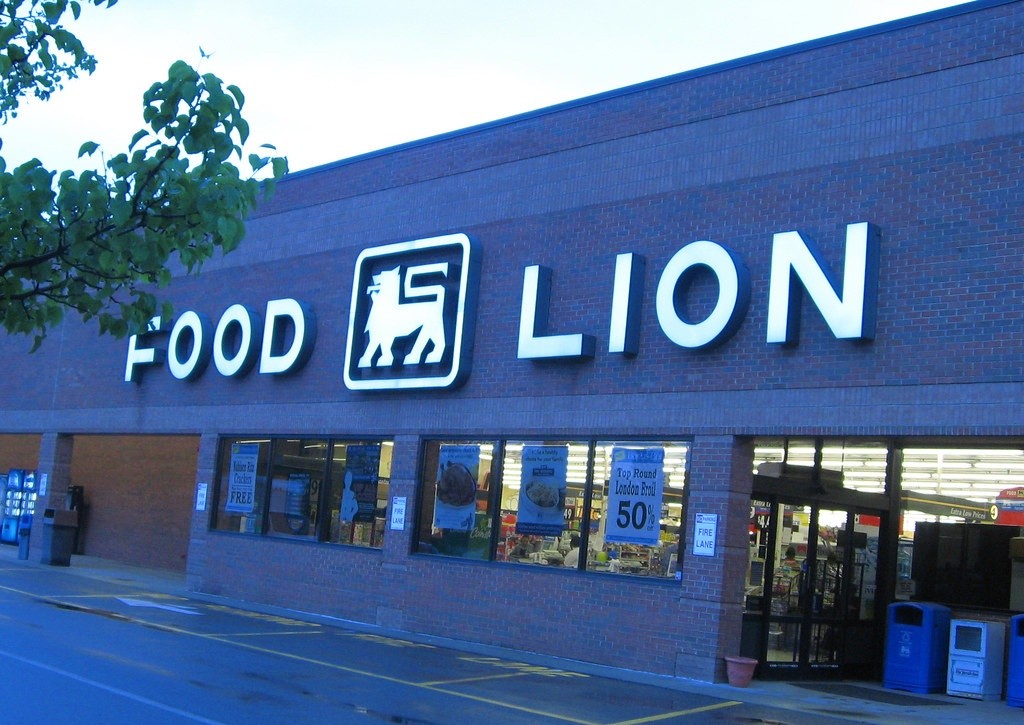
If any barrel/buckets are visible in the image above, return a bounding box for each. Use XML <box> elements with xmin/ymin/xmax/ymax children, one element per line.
<box><xmin>725</xmin><ymin>656</ymin><xmax>757</xmax><ymax>687</ymax></box>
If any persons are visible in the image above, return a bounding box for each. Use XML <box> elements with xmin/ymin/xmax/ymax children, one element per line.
<box><xmin>661</xmin><ymin>536</ymin><xmax>680</xmax><ymax>571</ymax></box>
<box><xmin>509</xmin><ymin>535</ymin><xmax>530</xmax><ymax>563</ymax></box>
<box><xmin>564</xmin><ymin>537</ymin><xmax>586</xmax><ymax>569</ymax></box>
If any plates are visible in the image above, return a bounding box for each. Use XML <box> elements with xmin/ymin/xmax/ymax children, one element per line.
<box><xmin>439</xmin><ymin>461</ymin><xmax>475</xmax><ymax>508</ymax></box>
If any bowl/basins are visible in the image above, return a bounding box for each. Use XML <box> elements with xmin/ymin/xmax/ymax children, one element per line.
<box><xmin>523</xmin><ymin>479</ymin><xmax>560</xmax><ymax>515</ymax></box>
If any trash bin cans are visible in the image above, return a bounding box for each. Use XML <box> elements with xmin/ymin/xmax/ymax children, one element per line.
<box><xmin>41</xmin><ymin>508</ymin><xmax>77</xmax><ymax>567</ymax></box>
<box><xmin>1007</xmin><ymin>613</ymin><xmax>1024</xmax><ymax>705</ymax></box>
<box><xmin>17</xmin><ymin>513</ymin><xmax>33</xmax><ymax>561</ymax></box>
<box><xmin>947</xmin><ymin>618</ymin><xmax>1006</xmax><ymax>701</ymax></box>
<box><xmin>881</xmin><ymin>600</ymin><xmax>953</xmax><ymax>695</ymax></box>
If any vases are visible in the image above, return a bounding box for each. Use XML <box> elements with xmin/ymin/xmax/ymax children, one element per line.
<box><xmin>723</xmin><ymin>657</ymin><xmax>758</xmax><ymax>688</ymax></box>
